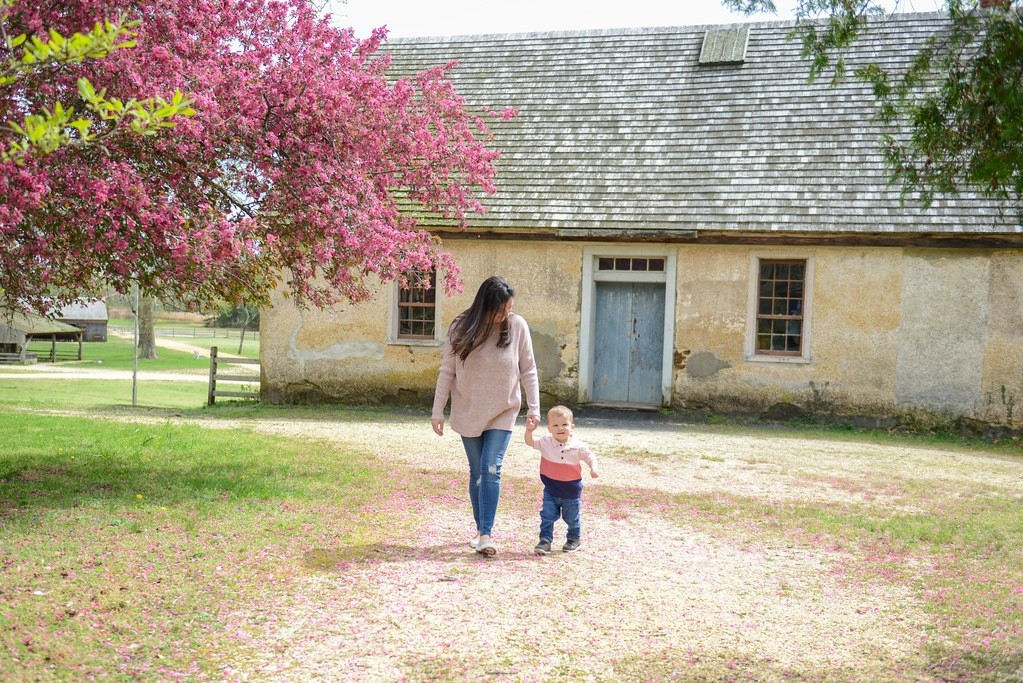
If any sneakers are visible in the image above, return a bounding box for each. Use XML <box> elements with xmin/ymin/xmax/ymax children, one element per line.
<box><xmin>563</xmin><ymin>538</ymin><xmax>581</xmax><ymax>552</ymax></box>
<box><xmin>534</xmin><ymin>541</ymin><xmax>552</xmax><ymax>554</ymax></box>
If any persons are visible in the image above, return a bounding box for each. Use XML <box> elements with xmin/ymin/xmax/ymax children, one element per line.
<box><xmin>525</xmin><ymin>405</ymin><xmax>599</xmax><ymax>555</ymax></box>
<box><xmin>431</xmin><ymin>276</ymin><xmax>541</xmax><ymax>554</ymax></box>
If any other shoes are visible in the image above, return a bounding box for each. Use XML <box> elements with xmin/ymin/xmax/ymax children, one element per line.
<box><xmin>476</xmin><ymin>542</ymin><xmax>496</xmax><ymax>555</ymax></box>
<box><xmin>470</xmin><ymin>538</ymin><xmax>479</xmax><ymax>547</ymax></box>
